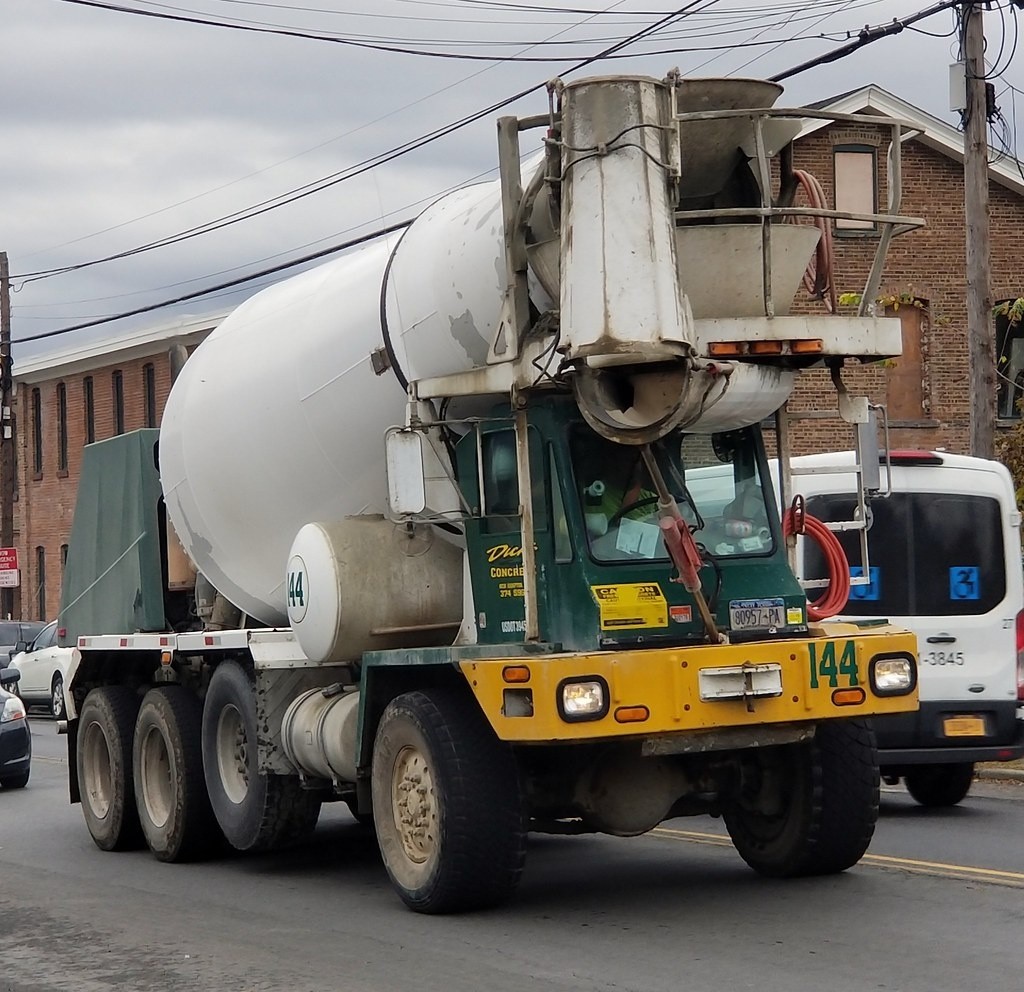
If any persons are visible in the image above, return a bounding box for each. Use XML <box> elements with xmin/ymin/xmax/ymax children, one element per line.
<box><xmin>580</xmin><ymin>461</ymin><xmax>659</xmax><ymax>539</ymax></box>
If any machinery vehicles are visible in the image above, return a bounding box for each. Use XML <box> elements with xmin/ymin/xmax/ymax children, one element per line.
<box><xmin>65</xmin><ymin>74</ymin><xmax>926</xmax><ymax>919</ymax></box>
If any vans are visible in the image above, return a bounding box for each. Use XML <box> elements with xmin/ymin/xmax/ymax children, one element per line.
<box><xmin>681</xmin><ymin>439</ymin><xmax>1024</xmax><ymax>810</ymax></box>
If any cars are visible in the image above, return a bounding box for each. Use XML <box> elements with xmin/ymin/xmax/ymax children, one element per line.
<box><xmin>0</xmin><ymin>619</ymin><xmax>75</xmax><ymax>787</ymax></box>
